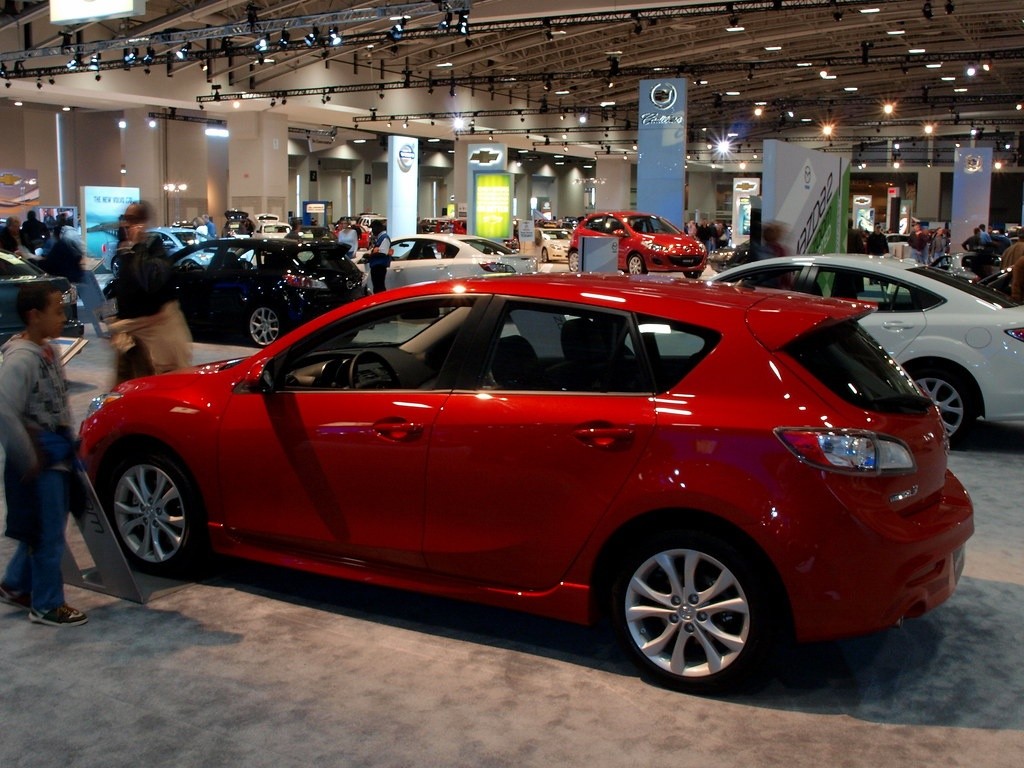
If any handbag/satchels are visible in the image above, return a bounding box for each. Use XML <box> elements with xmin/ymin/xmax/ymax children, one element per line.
<box><xmin>720</xmin><ymin>234</ymin><xmax>728</xmax><ymax>240</ymax></box>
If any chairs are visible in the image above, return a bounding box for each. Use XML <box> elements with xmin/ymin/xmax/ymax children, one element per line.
<box><xmin>220</xmin><ymin>252</ymin><xmax>243</xmax><ymax>270</ymax></box>
<box><xmin>831</xmin><ymin>272</ymin><xmax>857</xmax><ymax>299</ymax></box>
<box><xmin>488</xmin><ymin>315</ymin><xmax>629</xmax><ymax>392</ymax></box>
<box><xmin>420</xmin><ymin>246</ymin><xmax>435</xmax><ymax>258</ymax></box>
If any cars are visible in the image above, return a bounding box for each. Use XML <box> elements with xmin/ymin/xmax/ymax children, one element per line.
<box><xmin>709</xmin><ymin>239</ymin><xmax>750</xmax><ymax>273</ymax></box>
<box><xmin>568</xmin><ymin>211</ymin><xmax>708</xmax><ymax>280</ymax></box>
<box><xmin>884</xmin><ymin>232</ymin><xmax>910</xmax><ymax>254</ymax></box>
<box><xmin>350</xmin><ymin>232</ymin><xmax>540</xmax><ymax>295</ymax></box>
<box><xmin>101</xmin><ymin>212</ymin><xmax>518</xmax><ymax>349</ymax></box>
<box><xmin>74</xmin><ymin>272</ymin><xmax>976</xmax><ymax>694</ymax></box>
<box><xmin>534</xmin><ymin>228</ymin><xmax>571</xmax><ymax>262</ymax></box>
<box><xmin>705</xmin><ymin>252</ymin><xmax>1023</xmax><ymax>441</ymax></box>
<box><xmin>0</xmin><ymin>248</ymin><xmax>85</xmax><ymax>346</ymax></box>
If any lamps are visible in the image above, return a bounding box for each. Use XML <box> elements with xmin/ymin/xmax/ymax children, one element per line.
<box><xmin>0</xmin><ymin>0</ymin><xmax>1024</xmax><ymax>167</ymax></box>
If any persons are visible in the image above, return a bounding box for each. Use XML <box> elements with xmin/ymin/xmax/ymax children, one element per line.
<box><xmin>0</xmin><ymin>200</ymin><xmax>425</xmax><ymax>384</ymax></box>
<box><xmin>849</xmin><ymin>218</ymin><xmax>1024</xmax><ymax>305</ymax></box>
<box><xmin>757</xmin><ymin>220</ymin><xmax>793</xmax><ymax>290</ymax></box>
<box><xmin>0</xmin><ymin>280</ymin><xmax>89</xmax><ymax>627</ymax></box>
<box><xmin>513</xmin><ymin>213</ymin><xmax>730</xmax><ymax>257</ymax></box>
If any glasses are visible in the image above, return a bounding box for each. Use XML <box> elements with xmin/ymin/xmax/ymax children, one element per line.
<box><xmin>120</xmin><ymin>214</ymin><xmax>146</xmax><ymax>223</ymax></box>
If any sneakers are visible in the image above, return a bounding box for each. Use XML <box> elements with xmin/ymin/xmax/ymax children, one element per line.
<box><xmin>28</xmin><ymin>604</ymin><xmax>88</xmax><ymax>628</ymax></box>
<box><xmin>0</xmin><ymin>585</ymin><xmax>31</xmax><ymax>610</ymax></box>
